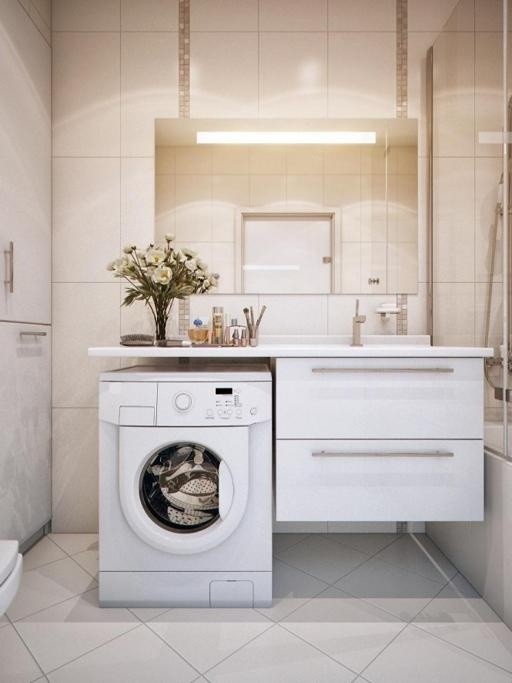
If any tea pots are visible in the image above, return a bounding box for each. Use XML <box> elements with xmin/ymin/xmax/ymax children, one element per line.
<box><xmin>154</xmin><ymin>117</ymin><xmax>419</xmax><ymax>296</ymax></box>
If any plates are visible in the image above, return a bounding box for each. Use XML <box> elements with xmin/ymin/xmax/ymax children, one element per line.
<box><xmin>1</xmin><ymin>0</ymin><xmax>54</xmax><ymax>555</ymax></box>
<box><xmin>275</xmin><ymin>355</ymin><xmax>484</xmax><ymax>522</ymax></box>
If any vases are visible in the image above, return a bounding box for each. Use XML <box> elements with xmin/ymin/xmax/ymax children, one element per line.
<box><xmin>107</xmin><ymin>232</ymin><xmax>217</xmax><ymax>339</ymax></box>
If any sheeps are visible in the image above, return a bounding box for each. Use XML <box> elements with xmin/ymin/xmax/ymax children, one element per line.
<box><xmin>352</xmin><ymin>301</ymin><xmax>366</xmax><ymax>346</ymax></box>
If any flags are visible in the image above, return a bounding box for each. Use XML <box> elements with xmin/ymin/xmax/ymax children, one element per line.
<box><xmin>145</xmin><ymin>297</ymin><xmax>175</xmax><ymax>345</ymax></box>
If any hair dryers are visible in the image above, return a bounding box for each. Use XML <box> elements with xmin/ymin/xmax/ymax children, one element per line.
<box><xmin>97</xmin><ymin>365</ymin><xmax>272</xmax><ymax>609</ymax></box>
